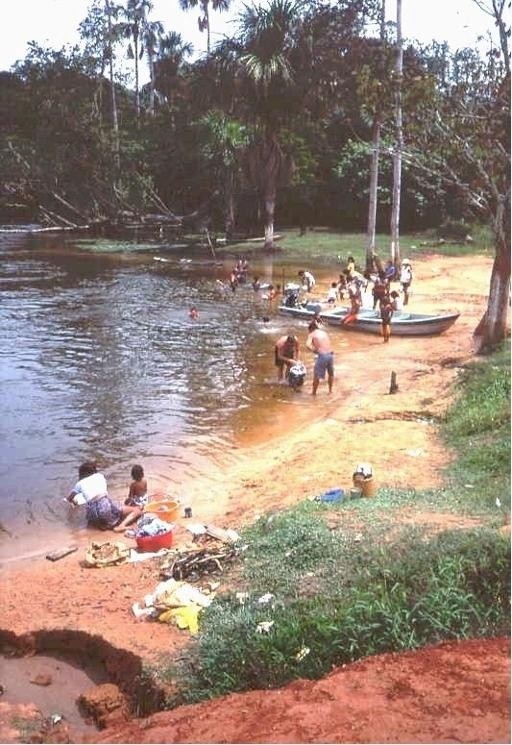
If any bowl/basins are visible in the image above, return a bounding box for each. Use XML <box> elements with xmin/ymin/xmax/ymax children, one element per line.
<box><xmin>136</xmin><ymin>501</ymin><xmax>177</xmax><ymax>552</ymax></box>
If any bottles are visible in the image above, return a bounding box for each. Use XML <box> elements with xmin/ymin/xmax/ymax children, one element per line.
<box><xmin>185</xmin><ymin>507</ymin><xmax>192</xmax><ymax>518</ymax></box>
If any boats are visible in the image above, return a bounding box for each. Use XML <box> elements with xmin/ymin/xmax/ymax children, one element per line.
<box><xmin>277</xmin><ymin>302</ymin><xmax>461</xmax><ymax>336</ymax></box>
<box><xmin>154</xmin><ymin>256</ymin><xmax>217</xmax><ymax>268</ymax></box>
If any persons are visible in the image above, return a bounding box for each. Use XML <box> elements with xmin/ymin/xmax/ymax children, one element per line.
<box><xmin>66</xmin><ymin>463</ymin><xmax>142</xmax><ymax>533</ymax></box>
<box><xmin>328</xmin><ymin>255</ymin><xmax>413</xmax><ymax>343</ymax></box>
<box><xmin>189</xmin><ymin>306</ymin><xmax>200</xmax><ymax>321</ymax></box>
<box><xmin>230</xmin><ymin>254</ymin><xmax>316</xmax><ymax>322</ymax></box>
<box><xmin>305</xmin><ymin>320</ymin><xmax>334</xmax><ymax>396</ymax></box>
<box><xmin>123</xmin><ymin>464</ymin><xmax>148</xmax><ymax>511</ymax></box>
<box><xmin>274</xmin><ymin>333</ymin><xmax>301</xmax><ymax>387</ymax></box>
